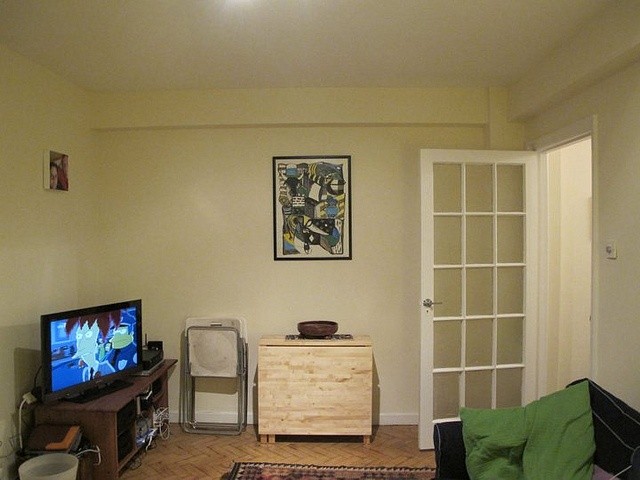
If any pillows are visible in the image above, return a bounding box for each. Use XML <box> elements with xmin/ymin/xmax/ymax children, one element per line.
<box><xmin>459</xmin><ymin>381</ymin><xmax>597</xmax><ymax>480</ymax></box>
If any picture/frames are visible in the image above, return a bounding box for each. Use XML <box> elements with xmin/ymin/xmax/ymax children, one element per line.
<box><xmin>44</xmin><ymin>148</ymin><xmax>70</xmax><ymax>193</ymax></box>
<box><xmin>272</xmin><ymin>155</ymin><xmax>353</xmax><ymax>261</ymax></box>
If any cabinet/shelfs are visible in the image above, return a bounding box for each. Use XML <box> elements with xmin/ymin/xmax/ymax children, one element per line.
<box><xmin>21</xmin><ymin>359</ymin><xmax>178</xmax><ymax>479</ymax></box>
<box><xmin>256</xmin><ymin>335</ymin><xmax>374</xmax><ymax>445</ymax></box>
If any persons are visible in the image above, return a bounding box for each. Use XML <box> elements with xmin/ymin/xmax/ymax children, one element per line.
<box><xmin>57</xmin><ymin>155</ymin><xmax>69</xmax><ymax>191</ymax></box>
<box><xmin>50</xmin><ymin>162</ymin><xmax>58</xmax><ymax>191</ymax></box>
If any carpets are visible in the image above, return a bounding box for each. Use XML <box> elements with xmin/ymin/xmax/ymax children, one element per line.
<box><xmin>225</xmin><ymin>461</ymin><xmax>436</xmax><ymax>480</ymax></box>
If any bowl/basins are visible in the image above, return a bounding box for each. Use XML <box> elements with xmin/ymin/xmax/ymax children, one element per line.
<box><xmin>297</xmin><ymin>320</ymin><xmax>339</xmax><ymax>339</ymax></box>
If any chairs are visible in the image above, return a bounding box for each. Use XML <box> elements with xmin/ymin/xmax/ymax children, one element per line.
<box><xmin>433</xmin><ymin>380</ymin><xmax>639</xmax><ymax>480</ymax></box>
<box><xmin>178</xmin><ymin>318</ymin><xmax>248</xmax><ymax>437</ymax></box>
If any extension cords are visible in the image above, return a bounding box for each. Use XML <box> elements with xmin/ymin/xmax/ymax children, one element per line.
<box><xmin>22</xmin><ymin>390</ymin><xmax>36</xmax><ymax>406</ymax></box>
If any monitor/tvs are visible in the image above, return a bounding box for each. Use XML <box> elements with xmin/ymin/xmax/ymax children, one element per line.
<box><xmin>40</xmin><ymin>298</ymin><xmax>142</xmax><ymax>404</ymax></box>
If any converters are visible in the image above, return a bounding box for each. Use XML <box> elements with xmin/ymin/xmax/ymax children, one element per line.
<box><xmin>30</xmin><ymin>386</ymin><xmax>43</xmax><ymax>399</ymax></box>
